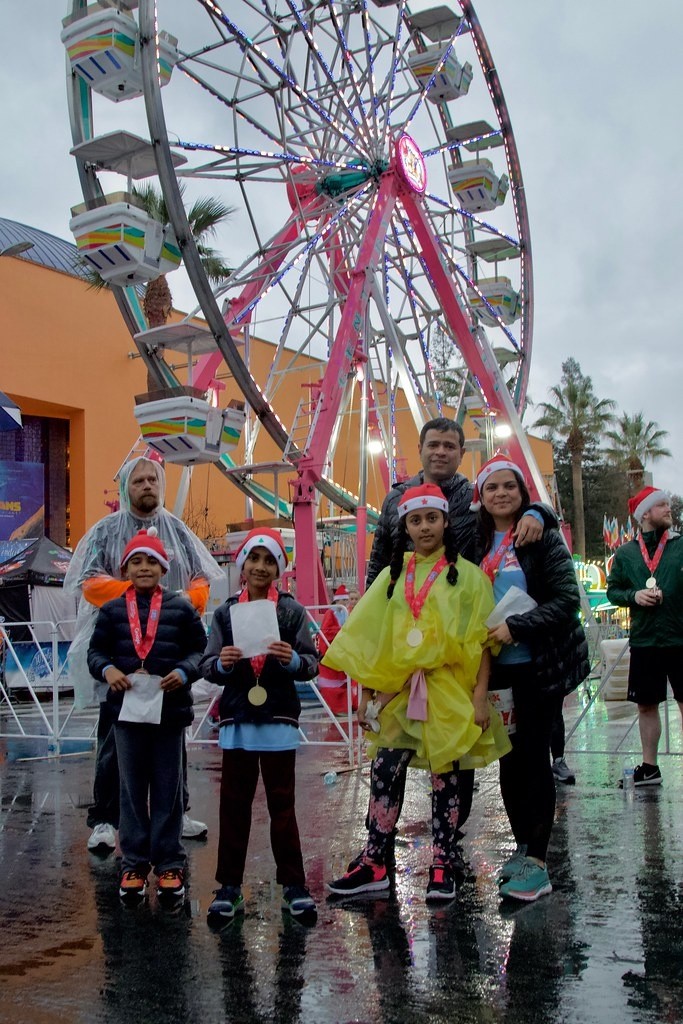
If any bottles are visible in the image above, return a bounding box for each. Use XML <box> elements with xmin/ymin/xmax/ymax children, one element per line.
<box><xmin>622</xmin><ymin>757</ymin><xmax>635</xmax><ymax>793</ymax></box>
<box><xmin>324</xmin><ymin>771</ymin><xmax>338</xmax><ymax>785</ymax></box>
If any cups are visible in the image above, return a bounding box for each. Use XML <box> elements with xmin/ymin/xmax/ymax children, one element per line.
<box><xmin>649</xmin><ymin>587</ymin><xmax>663</xmax><ymax>607</ymax></box>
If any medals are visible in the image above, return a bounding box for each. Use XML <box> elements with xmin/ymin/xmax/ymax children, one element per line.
<box><xmin>248</xmin><ymin>686</ymin><xmax>267</xmax><ymax>705</ymax></box>
<box><xmin>407</xmin><ymin>627</ymin><xmax>422</xmax><ymax>646</ymax></box>
<box><xmin>646</xmin><ymin>577</ymin><xmax>656</xmax><ymax>588</ymax></box>
<box><xmin>135</xmin><ymin>669</ymin><xmax>148</xmax><ymax>674</ymax></box>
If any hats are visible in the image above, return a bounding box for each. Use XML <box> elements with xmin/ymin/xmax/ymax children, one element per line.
<box><xmin>628</xmin><ymin>486</ymin><xmax>669</xmax><ymax>522</ymax></box>
<box><xmin>333</xmin><ymin>584</ymin><xmax>349</xmax><ymax>600</ymax></box>
<box><xmin>120</xmin><ymin>526</ymin><xmax>169</xmax><ymax>574</ymax></box>
<box><xmin>233</xmin><ymin>528</ymin><xmax>289</xmax><ymax>580</ymax></box>
<box><xmin>469</xmin><ymin>454</ymin><xmax>524</xmax><ymax>512</ymax></box>
<box><xmin>399</xmin><ymin>484</ymin><xmax>449</xmax><ymax>520</ymax></box>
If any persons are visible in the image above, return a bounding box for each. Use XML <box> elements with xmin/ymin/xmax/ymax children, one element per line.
<box><xmin>322</xmin><ymin>484</ymin><xmax>512</xmax><ymax>898</ymax></box>
<box><xmin>462</xmin><ymin>447</ymin><xmax>591</xmax><ymax>899</ymax></box>
<box><xmin>197</xmin><ymin>525</ymin><xmax>320</xmax><ymax>915</ymax></box>
<box><xmin>87</xmin><ymin>526</ymin><xmax>207</xmax><ymax>897</ymax></box>
<box><xmin>63</xmin><ymin>456</ymin><xmax>225</xmax><ymax>850</ymax></box>
<box><xmin>550</xmin><ymin>697</ymin><xmax>575</xmax><ymax>780</ymax></box>
<box><xmin>365</xmin><ymin>417</ymin><xmax>560</xmax><ymax>590</ymax></box>
<box><xmin>605</xmin><ymin>486</ymin><xmax>683</xmax><ymax>785</ymax></box>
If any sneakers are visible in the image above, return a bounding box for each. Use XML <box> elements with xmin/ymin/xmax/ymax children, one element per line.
<box><xmin>207</xmin><ymin>908</ymin><xmax>245</xmax><ymax>933</ymax></box>
<box><xmin>88</xmin><ymin>849</ymin><xmax>116</xmax><ymax>866</ymax></box>
<box><xmin>498</xmin><ymin>861</ymin><xmax>553</xmax><ymax>901</ymax></box>
<box><xmin>119</xmin><ymin>871</ymin><xmax>147</xmax><ymax>897</ymax></box>
<box><xmin>281</xmin><ymin>885</ymin><xmax>316</xmax><ymax>914</ymax></box>
<box><xmin>180</xmin><ymin>814</ymin><xmax>208</xmax><ymax>837</ymax></box>
<box><xmin>157</xmin><ymin>896</ymin><xmax>185</xmax><ymax>915</ymax></box>
<box><xmin>207</xmin><ymin>889</ymin><xmax>244</xmax><ymax>917</ymax></box>
<box><xmin>426</xmin><ymin>864</ymin><xmax>456</xmax><ymax>898</ymax></box>
<box><xmin>282</xmin><ymin>907</ymin><xmax>318</xmax><ymax>929</ymax></box>
<box><xmin>617</xmin><ymin>762</ymin><xmax>663</xmax><ymax>787</ymax></box>
<box><xmin>326</xmin><ymin>889</ymin><xmax>397</xmax><ymax>914</ymax></box>
<box><xmin>119</xmin><ymin>896</ymin><xmax>146</xmax><ymax>912</ymax></box>
<box><xmin>326</xmin><ymin>862</ymin><xmax>389</xmax><ymax>894</ymax></box>
<box><xmin>87</xmin><ymin>823</ymin><xmax>116</xmax><ymax>849</ymax></box>
<box><xmin>500</xmin><ymin>844</ymin><xmax>528</xmax><ymax>876</ymax></box>
<box><xmin>157</xmin><ymin>869</ymin><xmax>185</xmax><ymax>896</ymax></box>
<box><xmin>551</xmin><ymin>757</ymin><xmax>575</xmax><ymax>781</ymax></box>
